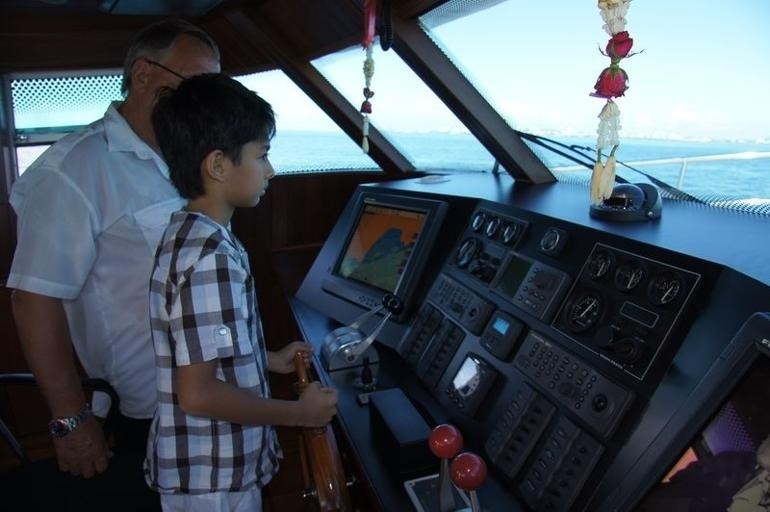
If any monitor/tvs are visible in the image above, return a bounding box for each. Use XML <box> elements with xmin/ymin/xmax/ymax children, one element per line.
<box><xmin>321</xmin><ymin>191</ymin><xmax>450</xmax><ymax>324</ymax></box>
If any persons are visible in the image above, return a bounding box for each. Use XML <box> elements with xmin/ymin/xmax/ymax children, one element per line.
<box><xmin>142</xmin><ymin>75</ymin><xmax>338</xmax><ymax>512</ymax></box>
<box><xmin>6</xmin><ymin>16</ymin><xmax>233</xmax><ymax>512</ymax></box>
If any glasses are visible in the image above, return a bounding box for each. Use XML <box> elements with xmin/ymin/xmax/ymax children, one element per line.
<box><xmin>144</xmin><ymin>59</ymin><xmax>186</xmax><ymax>80</ymax></box>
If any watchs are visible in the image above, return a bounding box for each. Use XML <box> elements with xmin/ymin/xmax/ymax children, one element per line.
<box><xmin>47</xmin><ymin>405</ymin><xmax>92</xmax><ymax>437</ymax></box>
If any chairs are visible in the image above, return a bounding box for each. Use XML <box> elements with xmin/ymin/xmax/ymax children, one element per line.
<box><xmin>0</xmin><ymin>370</ymin><xmax>162</xmax><ymax>511</ymax></box>
